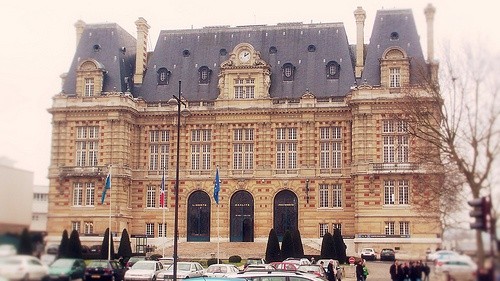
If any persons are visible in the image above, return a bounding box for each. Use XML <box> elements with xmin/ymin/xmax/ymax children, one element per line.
<box><xmin>333</xmin><ymin>260</ymin><xmax>342</xmax><ymax>281</ymax></box>
<box><xmin>389</xmin><ymin>259</ymin><xmax>445</xmax><ymax>281</ymax></box>
<box><xmin>311</xmin><ymin>257</ymin><xmax>315</xmax><ymax>264</ymax></box>
<box><xmin>327</xmin><ymin>259</ymin><xmax>335</xmax><ymax>281</ymax></box>
<box><xmin>319</xmin><ymin>261</ymin><xmax>327</xmax><ymax>274</ymax></box>
<box><xmin>355</xmin><ymin>259</ymin><xmax>368</xmax><ymax>281</ymax></box>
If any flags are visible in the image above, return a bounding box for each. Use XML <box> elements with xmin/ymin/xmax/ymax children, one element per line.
<box><xmin>213</xmin><ymin>165</ymin><xmax>221</xmax><ymax>205</ymax></box>
<box><xmin>159</xmin><ymin>167</ymin><xmax>166</xmax><ymax>208</ymax></box>
<box><xmin>100</xmin><ymin>166</ymin><xmax>113</xmax><ymax>205</ymax></box>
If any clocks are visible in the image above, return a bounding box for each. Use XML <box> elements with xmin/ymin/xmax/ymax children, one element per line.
<box><xmin>238</xmin><ymin>50</ymin><xmax>250</xmax><ymax>63</ymax></box>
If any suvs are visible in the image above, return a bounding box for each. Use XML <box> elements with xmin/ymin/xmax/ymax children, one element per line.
<box><xmin>380</xmin><ymin>248</ymin><xmax>395</xmax><ymax>260</ymax></box>
<box><xmin>361</xmin><ymin>248</ymin><xmax>377</xmax><ymax>261</ymax></box>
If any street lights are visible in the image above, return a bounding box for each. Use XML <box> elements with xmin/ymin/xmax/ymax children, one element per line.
<box><xmin>166</xmin><ymin>80</ymin><xmax>191</xmax><ymax>281</ymax></box>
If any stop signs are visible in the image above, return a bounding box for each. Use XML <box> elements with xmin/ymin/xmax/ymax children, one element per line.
<box><xmin>348</xmin><ymin>257</ymin><xmax>355</xmax><ymax>264</ymax></box>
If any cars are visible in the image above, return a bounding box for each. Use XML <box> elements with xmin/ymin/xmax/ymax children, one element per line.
<box><xmin>46</xmin><ymin>257</ymin><xmax>87</xmax><ymax>281</ymax></box>
<box><xmin>125</xmin><ymin>255</ymin><xmax>153</xmax><ymax>269</ymax></box>
<box><xmin>40</xmin><ymin>245</ymin><xmax>60</xmax><ymax>265</ymax></box>
<box><xmin>158</xmin><ymin>257</ymin><xmax>174</xmax><ymax>271</ymax></box>
<box><xmin>82</xmin><ymin>260</ymin><xmax>126</xmax><ymax>281</ymax></box>
<box><xmin>124</xmin><ymin>261</ymin><xmax>163</xmax><ymax>281</ymax></box>
<box><xmin>0</xmin><ymin>254</ymin><xmax>51</xmax><ymax>281</ymax></box>
<box><xmin>182</xmin><ymin>258</ymin><xmax>344</xmax><ymax>281</ymax></box>
<box><xmin>427</xmin><ymin>249</ymin><xmax>478</xmax><ymax>281</ymax></box>
<box><xmin>90</xmin><ymin>244</ymin><xmax>102</xmax><ymax>253</ymax></box>
<box><xmin>155</xmin><ymin>262</ymin><xmax>204</xmax><ymax>281</ymax></box>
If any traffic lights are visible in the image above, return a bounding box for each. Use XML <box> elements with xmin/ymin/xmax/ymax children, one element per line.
<box><xmin>467</xmin><ymin>196</ymin><xmax>487</xmax><ymax>232</ymax></box>
